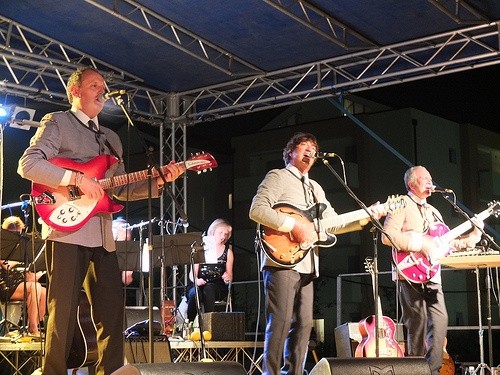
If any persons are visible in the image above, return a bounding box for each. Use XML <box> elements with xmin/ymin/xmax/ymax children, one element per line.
<box><xmin>111</xmin><ymin>218</ymin><xmax>135</xmax><ymax>284</ymax></box>
<box><xmin>0</xmin><ymin>216</ymin><xmax>47</xmax><ymax>338</ymax></box>
<box><xmin>17</xmin><ymin>67</ymin><xmax>184</xmax><ymax>375</ymax></box>
<box><xmin>249</xmin><ymin>133</ymin><xmax>387</xmax><ymax>375</ymax></box>
<box><xmin>182</xmin><ymin>219</ymin><xmax>233</xmax><ymax>339</ymax></box>
<box><xmin>381</xmin><ymin>166</ymin><xmax>485</xmax><ymax>375</ymax></box>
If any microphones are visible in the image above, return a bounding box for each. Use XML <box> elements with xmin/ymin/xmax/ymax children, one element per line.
<box><xmin>430</xmin><ymin>186</ymin><xmax>453</xmax><ymax>193</ymax></box>
<box><xmin>98</xmin><ymin>90</ymin><xmax>127</xmax><ymax>102</ymax></box>
<box><xmin>309</xmin><ymin>152</ymin><xmax>337</xmax><ymax>158</ymax></box>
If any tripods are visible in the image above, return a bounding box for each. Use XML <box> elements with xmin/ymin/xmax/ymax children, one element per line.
<box><xmin>0</xmin><ymin>227</ymin><xmax>46</xmax><ymax>343</ymax></box>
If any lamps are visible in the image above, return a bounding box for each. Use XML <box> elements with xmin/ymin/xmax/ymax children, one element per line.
<box><xmin>7</xmin><ymin>104</ymin><xmax>36</xmax><ymax>130</ymax></box>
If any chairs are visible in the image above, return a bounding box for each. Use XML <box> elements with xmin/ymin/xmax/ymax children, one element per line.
<box><xmin>200</xmin><ymin>277</ymin><xmax>232</xmax><ymax>313</ymax></box>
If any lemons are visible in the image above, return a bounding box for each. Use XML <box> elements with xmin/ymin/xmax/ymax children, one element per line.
<box><xmin>188</xmin><ymin>331</ymin><xmax>212</xmax><ymax>340</ymax></box>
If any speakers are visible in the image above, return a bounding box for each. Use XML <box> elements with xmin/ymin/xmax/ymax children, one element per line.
<box><xmin>200</xmin><ymin>312</ymin><xmax>245</xmax><ymax>341</ymax></box>
<box><xmin>308</xmin><ymin>356</ymin><xmax>432</xmax><ymax>375</ymax></box>
<box><xmin>110</xmin><ymin>361</ymin><xmax>249</xmax><ymax>375</ymax></box>
<box><xmin>334</xmin><ymin>322</ymin><xmax>404</xmax><ymax>358</ymax></box>
<box><xmin>123</xmin><ymin>335</ymin><xmax>173</xmax><ymax>365</ymax></box>
<box><xmin>124</xmin><ymin>306</ymin><xmax>162</xmax><ymax>329</ymax></box>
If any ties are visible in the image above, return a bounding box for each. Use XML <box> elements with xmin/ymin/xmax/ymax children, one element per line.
<box><xmin>88</xmin><ymin>120</ymin><xmax>94</xmax><ymax>130</ymax></box>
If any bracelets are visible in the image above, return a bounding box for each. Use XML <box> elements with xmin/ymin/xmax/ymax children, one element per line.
<box><xmin>75</xmin><ymin>172</ymin><xmax>82</xmax><ymax>187</ymax></box>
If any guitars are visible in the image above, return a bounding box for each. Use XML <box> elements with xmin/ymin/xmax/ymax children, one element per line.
<box><xmin>432</xmin><ymin>337</ymin><xmax>455</xmax><ymax>375</ymax></box>
<box><xmin>356</xmin><ymin>256</ymin><xmax>404</xmax><ymax>358</ymax></box>
<box><xmin>32</xmin><ymin>151</ymin><xmax>217</xmax><ymax>233</ymax></box>
<box><xmin>259</xmin><ymin>192</ymin><xmax>408</xmax><ymax>269</ymax></box>
<box><xmin>160</xmin><ymin>265</ymin><xmax>178</xmax><ymax>336</ymax></box>
<box><xmin>391</xmin><ymin>200</ymin><xmax>500</xmax><ymax>283</ymax></box>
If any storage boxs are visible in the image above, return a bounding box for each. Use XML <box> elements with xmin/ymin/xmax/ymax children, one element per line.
<box><xmin>200</xmin><ymin>312</ymin><xmax>245</xmax><ymax>341</ymax></box>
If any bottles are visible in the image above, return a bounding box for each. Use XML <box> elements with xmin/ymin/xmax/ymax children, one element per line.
<box><xmin>465</xmin><ymin>366</ymin><xmax>477</xmax><ymax>375</ymax></box>
<box><xmin>182</xmin><ymin>319</ymin><xmax>194</xmax><ymax>340</ymax></box>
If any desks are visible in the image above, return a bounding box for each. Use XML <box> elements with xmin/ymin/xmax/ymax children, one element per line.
<box><xmin>440</xmin><ymin>255</ymin><xmax>500</xmax><ymax>375</ymax></box>
